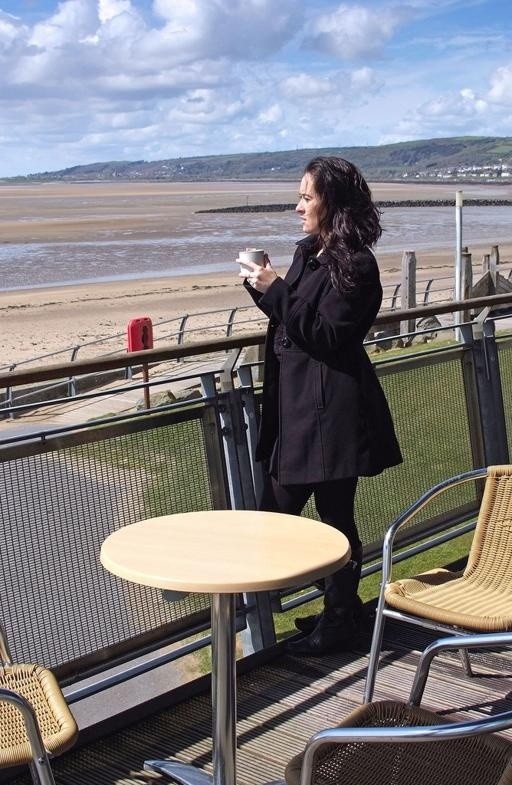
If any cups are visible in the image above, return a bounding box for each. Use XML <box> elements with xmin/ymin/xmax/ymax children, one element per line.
<box><xmin>239</xmin><ymin>249</ymin><xmax>264</xmax><ymax>273</ymax></box>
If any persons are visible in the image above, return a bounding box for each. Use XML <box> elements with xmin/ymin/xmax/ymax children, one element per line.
<box><xmin>235</xmin><ymin>157</ymin><xmax>403</xmax><ymax>656</ymax></box>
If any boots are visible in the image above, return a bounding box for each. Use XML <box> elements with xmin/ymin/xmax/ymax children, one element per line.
<box><xmin>287</xmin><ymin>543</ymin><xmax>363</xmax><ymax>655</ymax></box>
<box><xmin>295</xmin><ymin>577</ymin><xmax>365</xmax><ymax>631</ymax></box>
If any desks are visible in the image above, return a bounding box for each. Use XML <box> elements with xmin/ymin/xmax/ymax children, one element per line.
<box><xmin>100</xmin><ymin>509</ymin><xmax>352</xmax><ymax>785</ymax></box>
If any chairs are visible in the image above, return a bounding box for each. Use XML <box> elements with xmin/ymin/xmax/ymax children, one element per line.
<box><xmin>363</xmin><ymin>465</ymin><xmax>512</xmax><ymax>703</ymax></box>
<box><xmin>0</xmin><ymin>626</ymin><xmax>79</xmax><ymax>784</ymax></box>
<box><xmin>284</xmin><ymin>633</ymin><xmax>512</xmax><ymax>785</ymax></box>
